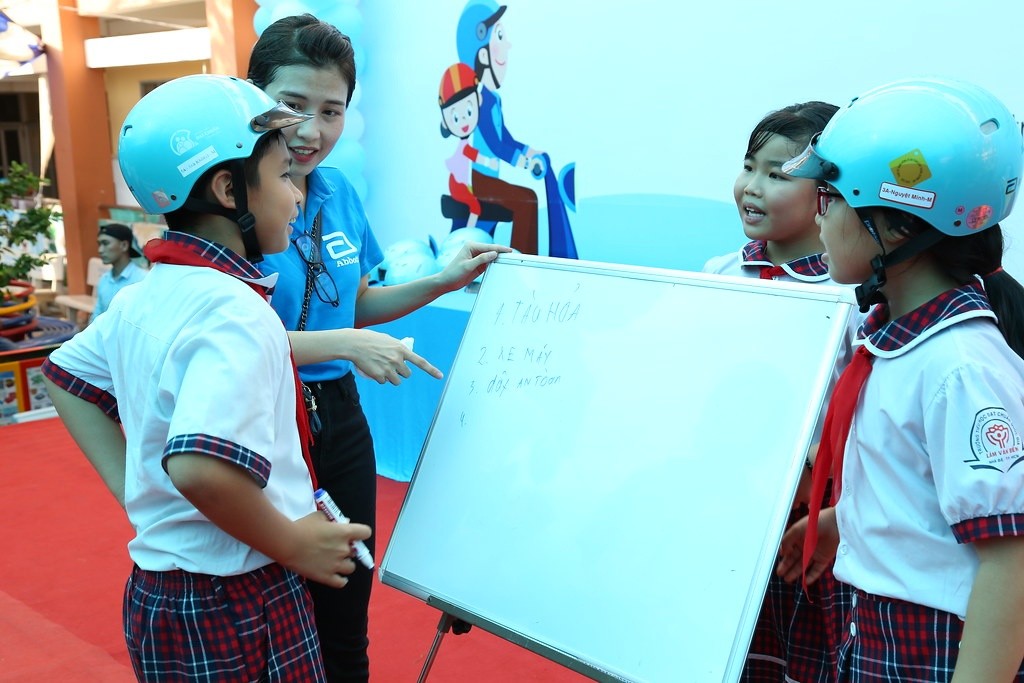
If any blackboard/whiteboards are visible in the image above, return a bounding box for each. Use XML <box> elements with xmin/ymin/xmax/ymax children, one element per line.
<box><xmin>378</xmin><ymin>246</ymin><xmax>856</xmax><ymax>683</ymax></box>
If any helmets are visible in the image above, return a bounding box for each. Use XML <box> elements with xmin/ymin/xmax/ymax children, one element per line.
<box><xmin>116</xmin><ymin>73</ymin><xmax>316</xmax><ymax>216</ymax></box>
<box><xmin>782</xmin><ymin>76</ymin><xmax>1024</xmax><ymax>236</ymax></box>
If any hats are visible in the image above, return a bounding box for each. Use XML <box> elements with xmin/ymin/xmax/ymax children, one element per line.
<box><xmin>98</xmin><ymin>224</ymin><xmax>142</xmax><ymax>258</ymax></box>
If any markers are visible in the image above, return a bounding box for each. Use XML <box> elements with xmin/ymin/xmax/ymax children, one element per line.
<box><xmin>314</xmin><ymin>488</ymin><xmax>379</xmax><ymax>573</ymax></box>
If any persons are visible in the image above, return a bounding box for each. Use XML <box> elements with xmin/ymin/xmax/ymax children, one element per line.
<box><xmin>246</xmin><ymin>13</ymin><xmax>512</xmax><ymax>683</ymax></box>
<box><xmin>776</xmin><ymin>77</ymin><xmax>1024</xmax><ymax>683</ymax></box>
<box><xmin>41</xmin><ymin>74</ymin><xmax>370</xmax><ymax>682</ymax></box>
<box><xmin>701</xmin><ymin>100</ymin><xmax>880</xmax><ymax>683</ymax></box>
<box><xmin>87</xmin><ymin>224</ymin><xmax>146</xmax><ymax>327</ymax></box>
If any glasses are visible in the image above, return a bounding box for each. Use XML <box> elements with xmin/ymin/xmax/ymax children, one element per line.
<box><xmin>817</xmin><ymin>186</ymin><xmax>846</xmax><ymax>216</ymax></box>
<box><xmin>290</xmin><ymin>230</ymin><xmax>340</xmax><ymax>308</ymax></box>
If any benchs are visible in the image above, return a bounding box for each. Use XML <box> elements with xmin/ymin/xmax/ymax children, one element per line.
<box><xmin>29</xmin><ymin>252</ymin><xmax>67</xmax><ymax>318</ymax></box>
<box><xmin>17</xmin><ymin>316</ymin><xmax>80</xmax><ymax>348</ymax></box>
<box><xmin>56</xmin><ymin>256</ymin><xmax>156</xmax><ymax>324</ymax></box>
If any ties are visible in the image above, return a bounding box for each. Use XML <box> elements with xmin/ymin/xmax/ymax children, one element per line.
<box><xmin>756</xmin><ymin>266</ymin><xmax>785</xmax><ymax>281</ymax></box>
<box><xmin>143</xmin><ymin>239</ymin><xmax>317</xmax><ymax>493</ymax></box>
<box><xmin>801</xmin><ymin>344</ymin><xmax>874</xmax><ymax>604</ymax></box>
